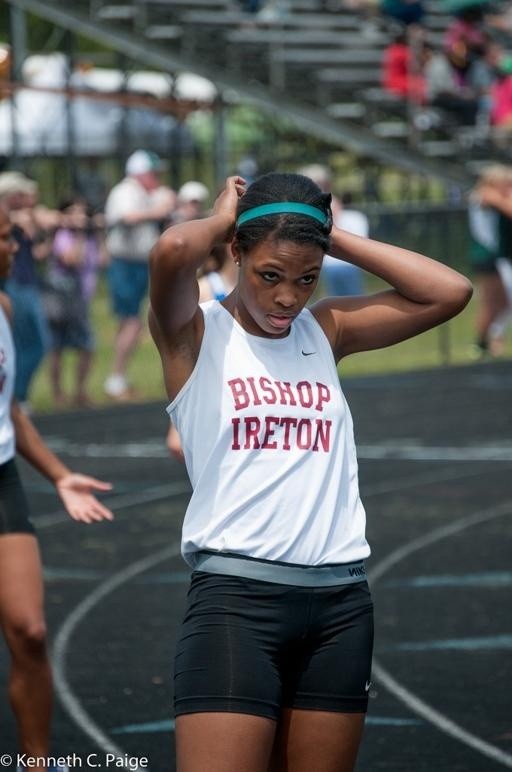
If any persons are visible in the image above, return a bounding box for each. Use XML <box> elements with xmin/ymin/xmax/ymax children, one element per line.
<box><xmin>1</xmin><ymin>148</ymin><xmax>224</xmax><ymax>417</ymax></box>
<box><xmin>318</xmin><ymin>0</ymin><xmax>511</xmax><ymax>362</ymax></box>
<box><xmin>145</xmin><ymin>171</ymin><xmax>475</xmax><ymax>772</ymax></box>
<box><xmin>1</xmin><ymin>198</ymin><xmax>119</xmax><ymax>771</ymax></box>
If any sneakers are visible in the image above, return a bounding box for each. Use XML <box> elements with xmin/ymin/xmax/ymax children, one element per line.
<box><xmin>102</xmin><ymin>372</ymin><xmax>130</xmax><ymax>406</ymax></box>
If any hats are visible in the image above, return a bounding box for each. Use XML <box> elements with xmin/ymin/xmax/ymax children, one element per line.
<box><xmin>123</xmin><ymin>148</ymin><xmax>169</xmax><ymax>174</ymax></box>
<box><xmin>178</xmin><ymin>181</ymin><xmax>210</xmax><ymax>202</ymax></box>
